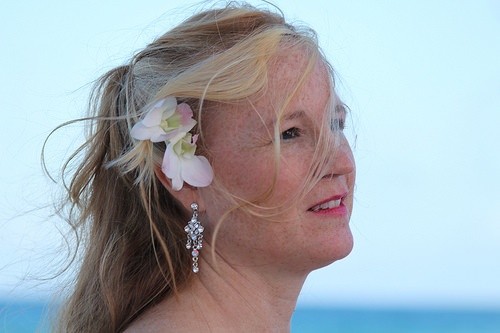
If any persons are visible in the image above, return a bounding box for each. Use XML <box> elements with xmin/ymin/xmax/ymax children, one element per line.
<box><xmin>41</xmin><ymin>3</ymin><xmax>358</xmax><ymax>332</ymax></box>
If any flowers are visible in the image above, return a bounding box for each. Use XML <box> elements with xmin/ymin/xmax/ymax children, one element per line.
<box><xmin>129</xmin><ymin>96</ymin><xmax>196</xmax><ymax>144</ymax></box>
<box><xmin>161</xmin><ymin>132</ymin><xmax>214</xmax><ymax>192</ymax></box>
